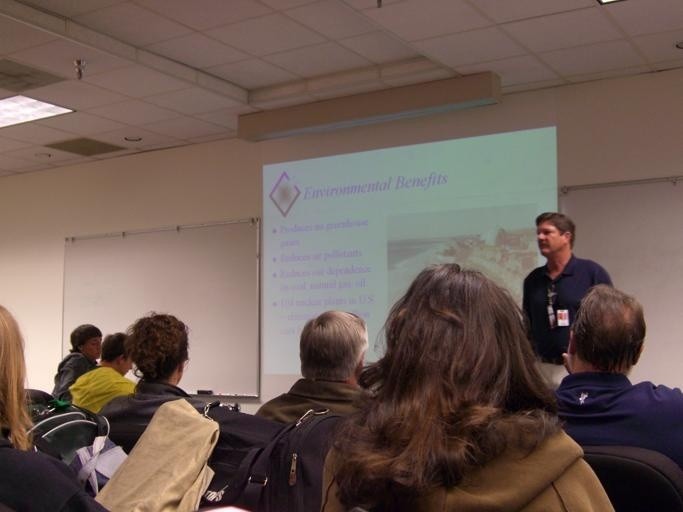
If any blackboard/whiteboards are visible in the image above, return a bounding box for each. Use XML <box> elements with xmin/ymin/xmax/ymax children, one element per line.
<box><xmin>62</xmin><ymin>218</ymin><xmax>260</xmax><ymax>397</ymax></box>
<box><xmin>558</xmin><ymin>175</ymin><xmax>683</xmax><ymax>392</ymax></box>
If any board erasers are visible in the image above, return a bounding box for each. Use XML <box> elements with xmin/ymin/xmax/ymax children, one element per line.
<box><xmin>197</xmin><ymin>390</ymin><xmax>212</xmax><ymax>394</ymax></box>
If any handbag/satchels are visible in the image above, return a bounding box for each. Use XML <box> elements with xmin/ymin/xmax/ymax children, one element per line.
<box><xmin>22</xmin><ymin>387</ymin><xmax>110</xmax><ymax>468</ymax></box>
<box><xmin>193</xmin><ymin>401</ymin><xmax>347</xmax><ymax>512</ymax></box>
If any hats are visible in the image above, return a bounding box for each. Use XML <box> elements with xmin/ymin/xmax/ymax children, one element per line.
<box><xmin>69</xmin><ymin>323</ymin><xmax>101</xmax><ymax>352</ymax></box>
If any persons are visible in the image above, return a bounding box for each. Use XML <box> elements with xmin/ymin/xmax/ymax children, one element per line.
<box><xmin>554</xmin><ymin>284</ymin><xmax>683</xmax><ymax>467</ymax></box>
<box><xmin>255</xmin><ymin>310</ymin><xmax>369</xmax><ymax>424</ymax></box>
<box><xmin>52</xmin><ymin>324</ymin><xmax>136</xmax><ymax>414</ymax></box>
<box><xmin>0</xmin><ymin>305</ymin><xmax>109</xmax><ymax>512</ymax></box>
<box><xmin>321</xmin><ymin>263</ymin><xmax>616</xmax><ymax>512</ymax></box>
<box><xmin>97</xmin><ymin>314</ymin><xmax>206</xmax><ymax>454</ymax></box>
<box><xmin>523</xmin><ymin>212</ymin><xmax>613</xmax><ymax>364</ymax></box>
